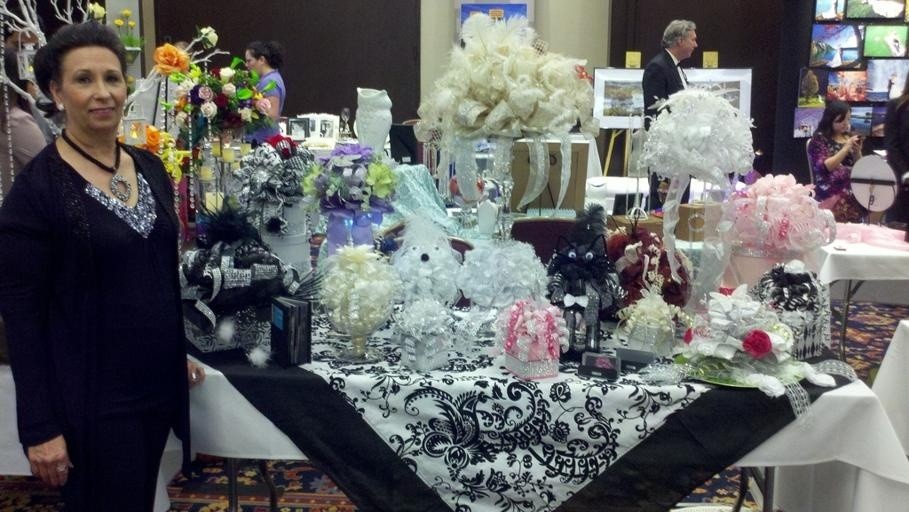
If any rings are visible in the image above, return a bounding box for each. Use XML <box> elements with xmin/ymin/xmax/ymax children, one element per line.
<box><xmin>191</xmin><ymin>372</ymin><xmax>200</xmax><ymax>383</ymax></box>
<box><xmin>56</xmin><ymin>465</ymin><xmax>67</xmax><ymax>473</ymax></box>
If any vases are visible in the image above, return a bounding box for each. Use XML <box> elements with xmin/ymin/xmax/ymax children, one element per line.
<box><xmin>124</xmin><ymin>46</ymin><xmax>141</xmax><ymax>64</ymax></box>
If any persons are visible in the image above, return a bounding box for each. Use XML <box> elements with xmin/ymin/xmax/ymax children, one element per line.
<box><xmin>0</xmin><ymin>46</ymin><xmax>62</xmax><ymax>201</ymax></box>
<box><xmin>883</xmin><ymin>73</ymin><xmax>909</xmax><ymax>243</ymax></box>
<box><xmin>0</xmin><ymin>21</ymin><xmax>206</xmax><ymax>512</ymax></box>
<box><xmin>245</xmin><ymin>41</ymin><xmax>286</xmax><ymax>150</ymax></box>
<box><xmin>807</xmin><ymin>101</ymin><xmax>885</xmax><ymax>225</ymax></box>
<box><xmin>643</xmin><ymin>20</ymin><xmax>698</xmax><ymax>210</ymax></box>
<box><xmin>3</xmin><ymin>7</ymin><xmax>44</xmax><ymax>116</ymax></box>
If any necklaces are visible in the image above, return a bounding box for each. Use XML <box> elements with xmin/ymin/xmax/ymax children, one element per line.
<box><xmin>61</xmin><ymin>128</ymin><xmax>132</xmax><ymax>202</ymax></box>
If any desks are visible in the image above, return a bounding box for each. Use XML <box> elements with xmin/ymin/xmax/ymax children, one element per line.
<box><xmin>803</xmin><ymin>223</ymin><xmax>909</xmax><ymax>362</ymax></box>
<box><xmin>187</xmin><ymin>306</ymin><xmax>909</xmax><ymax>512</ymax></box>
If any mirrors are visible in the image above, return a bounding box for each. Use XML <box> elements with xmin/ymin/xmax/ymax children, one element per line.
<box><xmin>849</xmin><ymin>155</ymin><xmax>898</xmax><ymax>226</ymax></box>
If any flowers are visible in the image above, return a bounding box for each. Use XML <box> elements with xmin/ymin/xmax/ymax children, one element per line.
<box><xmin>113</xmin><ymin>9</ymin><xmax>145</xmax><ymax>47</ymax></box>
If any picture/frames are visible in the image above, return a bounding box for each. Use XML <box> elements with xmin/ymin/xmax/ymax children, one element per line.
<box><xmin>278</xmin><ymin>112</ymin><xmax>339</xmax><ymax>143</ymax></box>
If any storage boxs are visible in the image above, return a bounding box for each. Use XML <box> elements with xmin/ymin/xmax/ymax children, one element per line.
<box><xmin>510</xmin><ymin>133</ymin><xmax>590</xmax><ymax>214</ymax></box>
